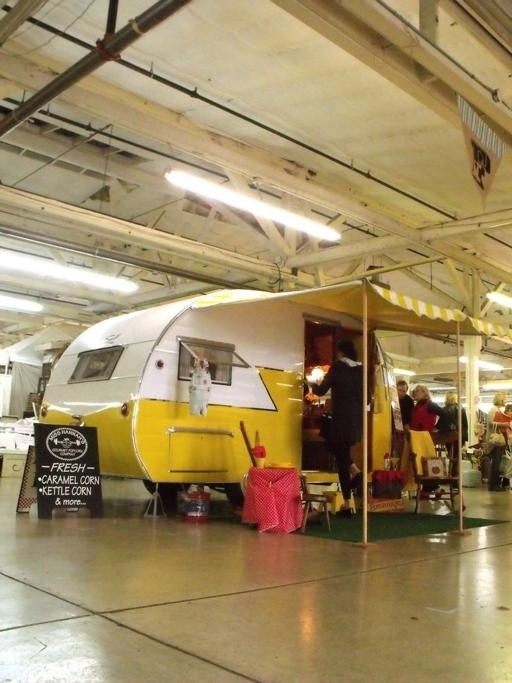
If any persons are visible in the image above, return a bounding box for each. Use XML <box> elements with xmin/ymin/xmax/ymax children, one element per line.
<box><xmin>301</xmin><ymin>337</ymin><xmax>369</xmax><ymax>515</ymax></box>
<box><xmin>475</xmin><ymin>391</ymin><xmax>511</xmax><ymax>492</ymax></box>
<box><xmin>433</xmin><ymin>390</ymin><xmax>468</xmax><ymax>489</ymax></box>
<box><xmin>408</xmin><ymin>384</ymin><xmax>446</xmax><ymax>500</ymax></box>
<box><xmin>394</xmin><ymin>380</ymin><xmax>412</xmax><ymax>422</ymax></box>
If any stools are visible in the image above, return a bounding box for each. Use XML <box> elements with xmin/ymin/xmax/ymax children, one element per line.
<box><xmin>300</xmin><ymin>493</ymin><xmax>332</xmax><ymax>535</ymax></box>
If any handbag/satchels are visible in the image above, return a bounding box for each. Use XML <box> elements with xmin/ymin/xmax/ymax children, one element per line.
<box><xmin>488</xmin><ymin>421</ymin><xmax>506</xmax><ymax>447</ymax></box>
<box><xmin>497</xmin><ymin>450</ymin><xmax>512</xmax><ymax>478</ymax></box>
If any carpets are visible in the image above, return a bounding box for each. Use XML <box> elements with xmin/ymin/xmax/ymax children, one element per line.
<box><xmin>232</xmin><ymin>508</ymin><xmax>511</xmax><ymax>544</ymax></box>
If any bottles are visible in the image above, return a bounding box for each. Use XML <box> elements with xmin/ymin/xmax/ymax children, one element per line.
<box><xmin>383</xmin><ymin>452</ymin><xmax>392</xmax><ymax>471</ymax></box>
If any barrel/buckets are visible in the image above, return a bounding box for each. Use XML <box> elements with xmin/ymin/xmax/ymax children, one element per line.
<box><xmin>186</xmin><ymin>487</ymin><xmax>213</xmax><ymax>522</ymax></box>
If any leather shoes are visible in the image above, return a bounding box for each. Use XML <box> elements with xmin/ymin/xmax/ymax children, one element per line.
<box><xmin>412</xmin><ymin>488</ymin><xmax>446</xmax><ymax>501</ymax></box>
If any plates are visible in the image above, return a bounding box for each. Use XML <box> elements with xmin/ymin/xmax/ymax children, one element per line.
<box><xmin>267</xmin><ymin>464</ymin><xmax>295</xmax><ymax>469</ymax></box>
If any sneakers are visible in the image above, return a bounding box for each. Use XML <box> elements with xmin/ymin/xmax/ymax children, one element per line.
<box><xmin>488</xmin><ymin>485</ymin><xmax>509</xmax><ymax>491</ymax></box>
<box><xmin>348</xmin><ymin>471</ymin><xmax>363</xmax><ymax>492</ymax></box>
<box><xmin>336</xmin><ymin>507</ymin><xmax>354</xmax><ymax>517</ymax></box>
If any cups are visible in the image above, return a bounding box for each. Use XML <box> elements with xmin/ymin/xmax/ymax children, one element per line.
<box><xmin>255</xmin><ymin>457</ymin><xmax>265</xmax><ymax>470</ymax></box>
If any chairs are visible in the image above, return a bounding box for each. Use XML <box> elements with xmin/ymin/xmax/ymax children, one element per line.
<box><xmin>409</xmin><ymin>430</ymin><xmax>465</xmax><ymax>515</ymax></box>
